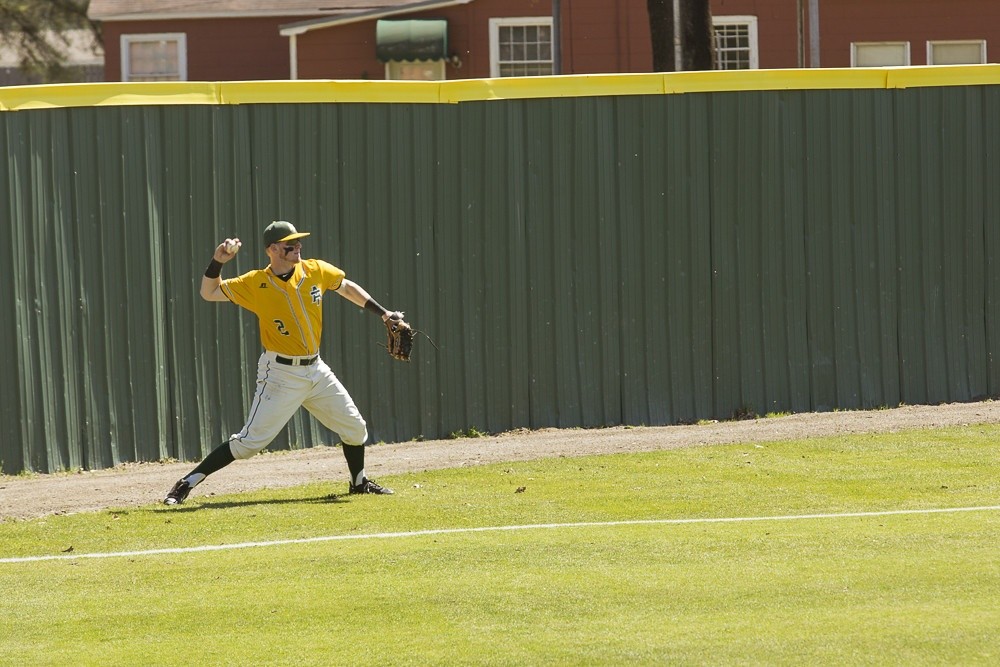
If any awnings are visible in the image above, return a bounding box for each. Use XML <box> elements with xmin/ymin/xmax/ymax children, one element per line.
<box><xmin>376</xmin><ymin>20</ymin><xmax>449</xmax><ymax>63</ymax></box>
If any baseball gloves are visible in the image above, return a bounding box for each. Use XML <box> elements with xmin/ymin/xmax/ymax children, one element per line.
<box><xmin>386</xmin><ymin>314</ymin><xmax>413</xmax><ymax>363</ymax></box>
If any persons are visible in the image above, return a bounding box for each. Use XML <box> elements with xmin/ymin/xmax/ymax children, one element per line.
<box><xmin>164</xmin><ymin>220</ymin><xmax>413</xmax><ymax>505</ymax></box>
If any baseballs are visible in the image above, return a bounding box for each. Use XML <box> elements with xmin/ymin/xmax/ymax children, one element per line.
<box><xmin>225</xmin><ymin>241</ymin><xmax>240</xmax><ymax>255</ymax></box>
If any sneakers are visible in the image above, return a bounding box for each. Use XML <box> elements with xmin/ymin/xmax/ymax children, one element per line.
<box><xmin>163</xmin><ymin>478</ymin><xmax>191</xmax><ymax>506</ymax></box>
<box><xmin>348</xmin><ymin>477</ymin><xmax>394</xmax><ymax>495</ymax></box>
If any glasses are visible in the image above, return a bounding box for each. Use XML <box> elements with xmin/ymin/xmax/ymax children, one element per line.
<box><xmin>288</xmin><ymin>237</ymin><xmax>301</xmax><ymax>245</ymax></box>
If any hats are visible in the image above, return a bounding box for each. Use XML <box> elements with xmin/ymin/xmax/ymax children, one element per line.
<box><xmin>262</xmin><ymin>221</ymin><xmax>310</xmax><ymax>246</ymax></box>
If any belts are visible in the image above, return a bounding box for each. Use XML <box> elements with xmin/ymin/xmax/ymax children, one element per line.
<box><xmin>274</xmin><ymin>355</ymin><xmax>320</xmax><ymax>366</ymax></box>
